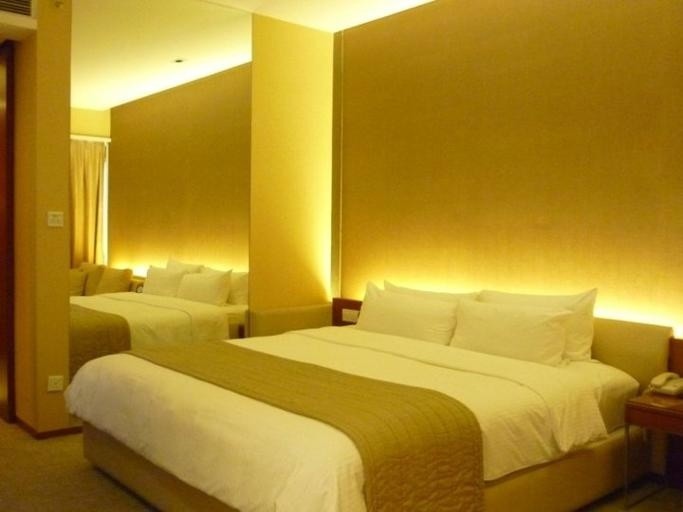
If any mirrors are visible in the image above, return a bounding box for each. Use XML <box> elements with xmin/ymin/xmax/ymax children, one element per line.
<box><xmin>72</xmin><ymin>2</ymin><xmax>249</xmax><ymax>387</ymax></box>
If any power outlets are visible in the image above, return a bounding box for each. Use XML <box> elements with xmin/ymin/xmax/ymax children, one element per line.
<box><xmin>45</xmin><ymin>375</ymin><xmax>64</xmax><ymax>393</ymax></box>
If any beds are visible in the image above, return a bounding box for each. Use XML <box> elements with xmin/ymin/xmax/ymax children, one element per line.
<box><xmin>62</xmin><ymin>276</ymin><xmax>683</xmax><ymax>512</ymax></box>
<box><xmin>67</xmin><ymin>262</ymin><xmax>251</xmax><ymax>382</ymax></box>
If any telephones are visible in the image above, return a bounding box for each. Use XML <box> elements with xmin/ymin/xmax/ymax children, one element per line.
<box><xmin>648</xmin><ymin>372</ymin><xmax>683</xmax><ymax>396</ymax></box>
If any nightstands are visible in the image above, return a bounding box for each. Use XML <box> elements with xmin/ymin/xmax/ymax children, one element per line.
<box><xmin>624</xmin><ymin>392</ymin><xmax>682</xmax><ymax>511</ymax></box>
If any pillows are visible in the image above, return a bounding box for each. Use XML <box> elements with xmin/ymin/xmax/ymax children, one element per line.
<box><xmin>69</xmin><ymin>263</ymin><xmax>248</xmax><ymax>304</ymax></box>
<box><xmin>356</xmin><ymin>281</ymin><xmax>597</xmax><ymax>367</ymax></box>
